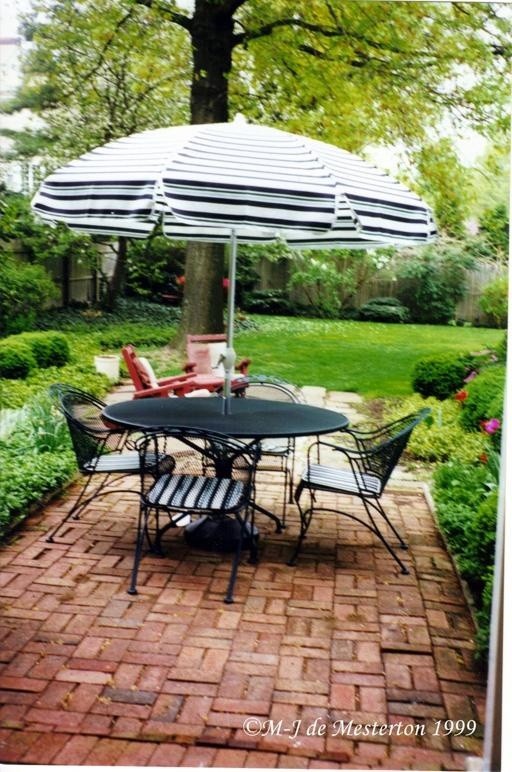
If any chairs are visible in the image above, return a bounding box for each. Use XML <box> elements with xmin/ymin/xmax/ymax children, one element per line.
<box><xmin>45</xmin><ymin>332</ymin><xmax>425</xmax><ymax>605</ymax></box>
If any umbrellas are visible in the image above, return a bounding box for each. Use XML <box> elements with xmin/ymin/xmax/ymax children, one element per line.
<box><xmin>30</xmin><ymin>112</ymin><xmax>439</xmax><ymax>416</ymax></box>
